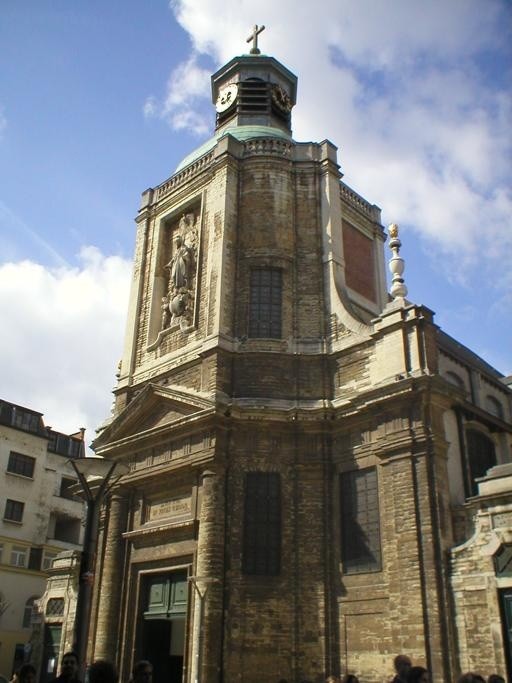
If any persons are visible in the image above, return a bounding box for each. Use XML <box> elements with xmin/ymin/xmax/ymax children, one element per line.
<box><xmin>326</xmin><ymin>656</ymin><xmax>505</xmax><ymax>682</ymax></box>
<box><xmin>165</xmin><ymin>234</ymin><xmax>192</xmax><ymax>290</ymax></box>
<box><xmin>0</xmin><ymin>653</ymin><xmax>153</xmax><ymax>683</ymax></box>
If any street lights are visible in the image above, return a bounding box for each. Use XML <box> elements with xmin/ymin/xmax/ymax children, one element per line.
<box><xmin>65</xmin><ymin>458</ymin><xmax>132</xmax><ymax>683</ymax></box>
